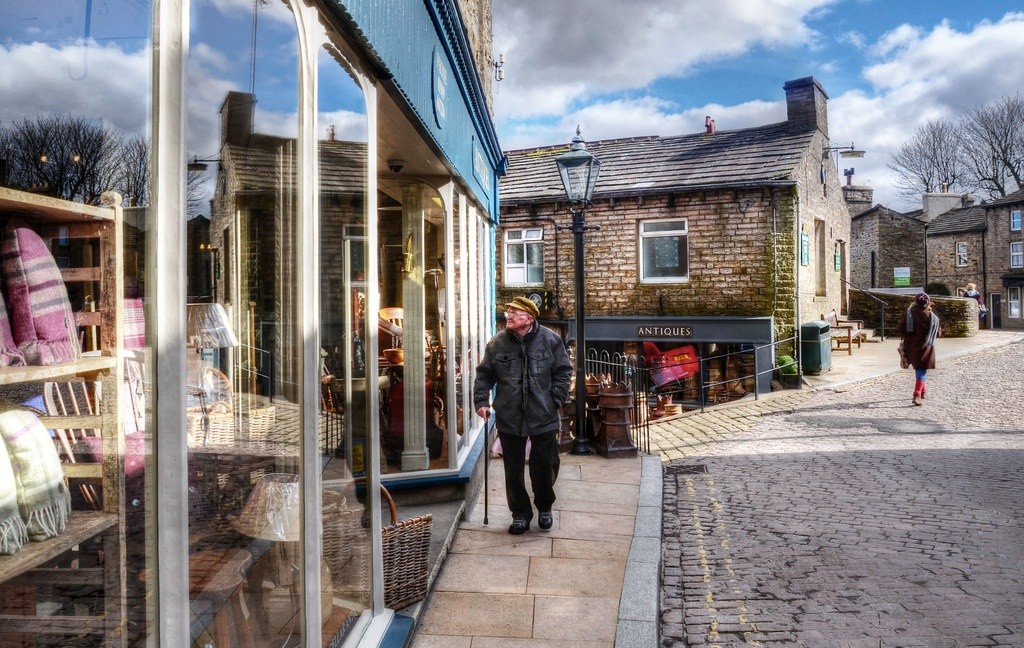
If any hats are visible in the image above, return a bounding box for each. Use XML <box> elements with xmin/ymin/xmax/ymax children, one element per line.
<box><xmin>965</xmin><ymin>283</ymin><xmax>974</xmax><ymax>291</ymax></box>
<box><xmin>504</xmin><ymin>296</ymin><xmax>540</xmax><ymax>319</ymax></box>
<box><xmin>915</xmin><ymin>292</ymin><xmax>930</xmax><ymax>305</ymax></box>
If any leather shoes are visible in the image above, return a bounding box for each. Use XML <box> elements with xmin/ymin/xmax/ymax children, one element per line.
<box><xmin>509</xmin><ymin>517</ymin><xmax>530</xmax><ymax>535</ymax></box>
<box><xmin>539</xmin><ymin>510</ymin><xmax>553</xmax><ymax>528</ymax></box>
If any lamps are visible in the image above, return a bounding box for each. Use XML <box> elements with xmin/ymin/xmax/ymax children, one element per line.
<box><xmin>233</xmin><ymin>471</ymin><xmax>302</xmax><ymax>648</ymax></box>
<box><xmin>187</xmin><ymin>154</ymin><xmax>222</xmax><ymax>170</ymax></box>
<box><xmin>187</xmin><ymin>304</ymin><xmax>239</xmax><ymax>522</ymax></box>
<box><xmin>493</xmin><ymin>51</ymin><xmax>505</xmax><ymax>80</ymax></box>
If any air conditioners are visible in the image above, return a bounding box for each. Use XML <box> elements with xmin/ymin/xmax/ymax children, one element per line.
<box><xmin>522</xmin><ymin>290</ymin><xmax>553</xmax><ymax>311</ymax></box>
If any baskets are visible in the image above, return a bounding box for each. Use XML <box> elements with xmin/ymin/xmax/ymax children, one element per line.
<box><xmin>186</xmin><ymin>368</ymin><xmax>432</xmax><ymax>610</ymax></box>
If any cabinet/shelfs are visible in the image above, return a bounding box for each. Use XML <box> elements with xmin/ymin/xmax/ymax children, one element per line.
<box><xmin>1</xmin><ymin>186</ymin><xmax>128</xmax><ymax>648</ymax></box>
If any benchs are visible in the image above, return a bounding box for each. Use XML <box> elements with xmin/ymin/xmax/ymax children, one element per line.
<box><xmin>821</xmin><ymin>308</ymin><xmax>863</xmax><ymax>355</ymax></box>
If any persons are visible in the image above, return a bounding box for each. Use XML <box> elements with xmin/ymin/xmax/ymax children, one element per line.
<box><xmin>473</xmin><ymin>297</ymin><xmax>573</xmax><ymax>537</ymax></box>
<box><xmin>898</xmin><ymin>293</ymin><xmax>939</xmax><ymax>405</ymax></box>
<box><xmin>963</xmin><ymin>283</ymin><xmax>981</xmax><ymax>300</ymax></box>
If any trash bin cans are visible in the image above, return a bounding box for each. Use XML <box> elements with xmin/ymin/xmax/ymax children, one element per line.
<box><xmin>801</xmin><ymin>321</ymin><xmax>833</xmax><ymax>376</ymax></box>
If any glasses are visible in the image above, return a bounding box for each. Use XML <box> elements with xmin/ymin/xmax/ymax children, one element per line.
<box><xmin>504</xmin><ymin>311</ymin><xmax>528</xmax><ymax>318</ymax></box>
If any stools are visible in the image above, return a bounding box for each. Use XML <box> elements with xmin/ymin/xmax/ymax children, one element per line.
<box><xmin>136</xmin><ymin>548</ymin><xmax>255</xmax><ymax>648</ymax></box>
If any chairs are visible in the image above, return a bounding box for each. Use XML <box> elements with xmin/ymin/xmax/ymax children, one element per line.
<box><xmin>42</xmin><ymin>377</ymin><xmax>270</xmax><ymax>639</ymax></box>
<box><xmin>378</xmin><ymin>308</ymin><xmax>438</xmax><ymax>394</ymax></box>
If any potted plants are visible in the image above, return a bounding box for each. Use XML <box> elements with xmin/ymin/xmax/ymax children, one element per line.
<box><xmin>776</xmin><ymin>356</ymin><xmax>802</xmax><ymax>388</ymax></box>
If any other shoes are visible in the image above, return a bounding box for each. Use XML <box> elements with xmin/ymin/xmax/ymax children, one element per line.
<box><xmin>898</xmin><ymin>337</ymin><xmax>910</xmax><ymax>369</ymax></box>
<box><xmin>912</xmin><ymin>396</ymin><xmax>922</xmax><ymax>406</ymax></box>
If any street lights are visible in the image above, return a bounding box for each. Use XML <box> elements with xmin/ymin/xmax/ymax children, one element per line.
<box><xmin>556</xmin><ymin>124</ymin><xmax>603</xmax><ymax>454</ymax></box>
